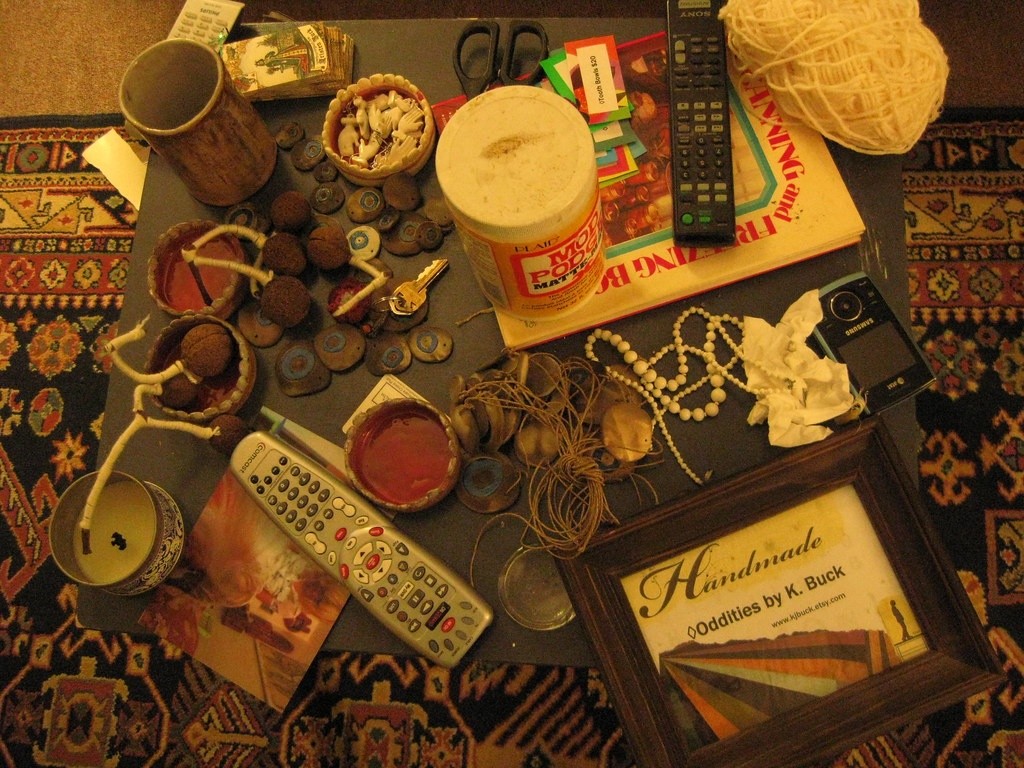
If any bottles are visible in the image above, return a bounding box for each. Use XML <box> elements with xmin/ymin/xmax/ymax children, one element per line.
<box><xmin>436</xmin><ymin>85</ymin><xmax>607</xmax><ymax>322</ymax></box>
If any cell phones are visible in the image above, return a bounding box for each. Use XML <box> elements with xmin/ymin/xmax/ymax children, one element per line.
<box><xmin>809</xmin><ymin>271</ymin><xmax>938</xmax><ymax>418</ymax></box>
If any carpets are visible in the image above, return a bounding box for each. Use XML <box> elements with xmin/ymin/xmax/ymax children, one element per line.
<box><xmin>0</xmin><ymin>104</ymin><xmax>1024</xmax><ymax>768</ymax></box>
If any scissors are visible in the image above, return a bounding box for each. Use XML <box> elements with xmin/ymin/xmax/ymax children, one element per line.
<box><xmin>450</xmin><ymin>17</ymin><xmax>551</xmax><ymax>111</ymax></box>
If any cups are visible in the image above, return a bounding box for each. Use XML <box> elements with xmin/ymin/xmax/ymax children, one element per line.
<box><xmin>119</xmin><ymin>39</ymin><xmax>278</xmax><ymax>207</ymax></box>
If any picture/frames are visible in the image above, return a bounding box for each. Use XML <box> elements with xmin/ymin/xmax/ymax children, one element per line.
<box><xmin>552</xmin><ymin>413</ymin><xmax>1006</xmax><ymax>768</ymax></box>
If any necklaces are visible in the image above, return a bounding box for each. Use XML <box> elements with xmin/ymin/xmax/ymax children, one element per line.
<box><xmin>583</xmin><ymin>307</ymin><xmax>797</xmax><ymax>483</ymax></box>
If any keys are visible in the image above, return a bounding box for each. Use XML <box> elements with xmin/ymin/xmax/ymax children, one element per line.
<box><xmin>388</xmin><ymin>257</ymin><xmax>451</xmax><ymax>317</ymax></box>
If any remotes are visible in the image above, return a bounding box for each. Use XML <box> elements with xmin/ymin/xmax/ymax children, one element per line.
<box><xmin>229</xmin><ymin>430</ymin><xmax>494</xmax><ymax>667</ymax></box>
<box><xmin>666</xmin><ymin>0</ymin><xmax>738</xmax><ymax>242</ymax></box>
<box><xmin>167</xmin><ymin>0</ymin><xmax>246</xmax><ymax>56</ymax></box>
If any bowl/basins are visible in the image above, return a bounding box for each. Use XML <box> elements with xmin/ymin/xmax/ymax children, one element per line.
<box><xmin>146</xmin><ymin>218</ymin><xmax>246</xmax><ymax>321</ymax></box>
<box><xmin>48</xmin><ymin>470</ymin><xmax>184</xmax><ymax>597</ymax></box>
<box><xmin>321</xmin><ymin>73</ymin><xmax>435</xmax><ymax>187</ymax></box>
<box><xmin>345</xmin><ymin>398</ymin><xmax>462</xmax><ymax>513</ymax></box>
<box><xmin>142</xmin><ymin>313</ymin><xmax>256</xmax><ymax>424</ymax></box>
